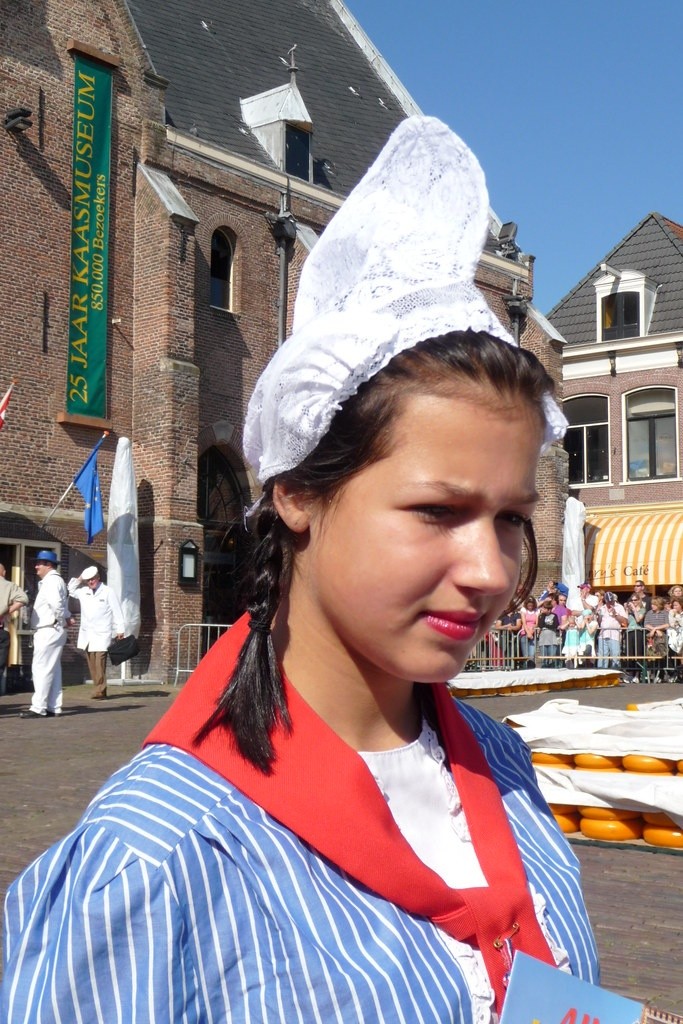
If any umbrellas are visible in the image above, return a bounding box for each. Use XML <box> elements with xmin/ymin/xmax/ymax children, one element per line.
<box><xmin>100</xmin><ymin>435</ymin><xmax>142</xmax><ymax>680</ymax></box>
<box><xmin>562</xmin><ymin>495</ymin><xmax>588</xmax><ymax>616</ymax></box>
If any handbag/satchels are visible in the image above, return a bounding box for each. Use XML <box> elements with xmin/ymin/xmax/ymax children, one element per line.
<box><xmin>108</xmin><ymin>635</ymin><xmax>140</xmax><ymax>667</ymax></box>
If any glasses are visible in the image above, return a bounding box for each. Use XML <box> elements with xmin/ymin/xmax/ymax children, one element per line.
<box><xmin>633</xmin><ymin>584</ymin><xmax>643</xmax><ymax>587</ymax></box>
<box><xmin>630</xmin><ymin>599</ymin><xmax>639</xmax><ymax>602</ymax></box>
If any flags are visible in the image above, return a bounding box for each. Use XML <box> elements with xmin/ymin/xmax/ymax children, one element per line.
<box><xmin>69</xmin><ymin>438</ymin><xmax>104</xmax><ymax>544</ymax></box>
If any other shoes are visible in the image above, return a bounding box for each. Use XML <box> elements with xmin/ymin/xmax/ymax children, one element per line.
<box><xmin>92</xmin><ymin>689</ymin><xmax>107</xmax><ymax>699</ymax></box>
<box><xmin>620</xmin><ymin>667</ymin><xmax>682</xmax><ymax>684</ymax></box>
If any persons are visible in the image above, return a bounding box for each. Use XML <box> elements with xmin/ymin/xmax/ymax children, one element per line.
<box><xmin>19</xmin><ymin>550</ymin><xmax>76</xmax><ymax>719</ymax></box>
<box><xmin>0</xmin><ymin>564</ymin><xmax>29</xmax><ymax>689</ymax></box>
<box><xmin>0</xmin><ymin>112</ymin><xmax>602</xmax><ymax>1024</ymax></box>
<box><xmin>467</xmin><ymin>577</ymin><xmax>683</xmax><ymax>676</ymax></box>
<box><xmin>64</xmin><ymin>564</ymin><xmax>123</xmax><ymax>701</ymax></box>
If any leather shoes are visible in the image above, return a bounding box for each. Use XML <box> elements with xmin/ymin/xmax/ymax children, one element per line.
<box><xmin>45</xmin><ymin>708</ymin><xmax>60</xmax><ymax>717</ymax></box>
<box><xmin>19</xmin><ymin>710</ymin><xmax>48</xmax><ymax>718</ymax></box>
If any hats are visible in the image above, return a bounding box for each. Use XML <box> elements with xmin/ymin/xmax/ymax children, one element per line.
<box><xmin>32</xmin><ymin>550</ymin><xmax>60</xmax><ymax>565</ymax></box>
<box><xmin>577</xmin><ymin>583</ymin><xmax>592</xmax><ymax>589</ymax></box>
<box><xmin>81</xmin><ymin>566</ymin><xmax>99</xmax><ymax>580</ymax></box>
<box><xmin>604</xmin><ymin>592</ymin><xmax>614</xmax><ymax>603</ymax></box>
<box><xmin>241</xmin><ymin>116</ymin><xmax>569</xmax><ymax>482</ymax></box>
